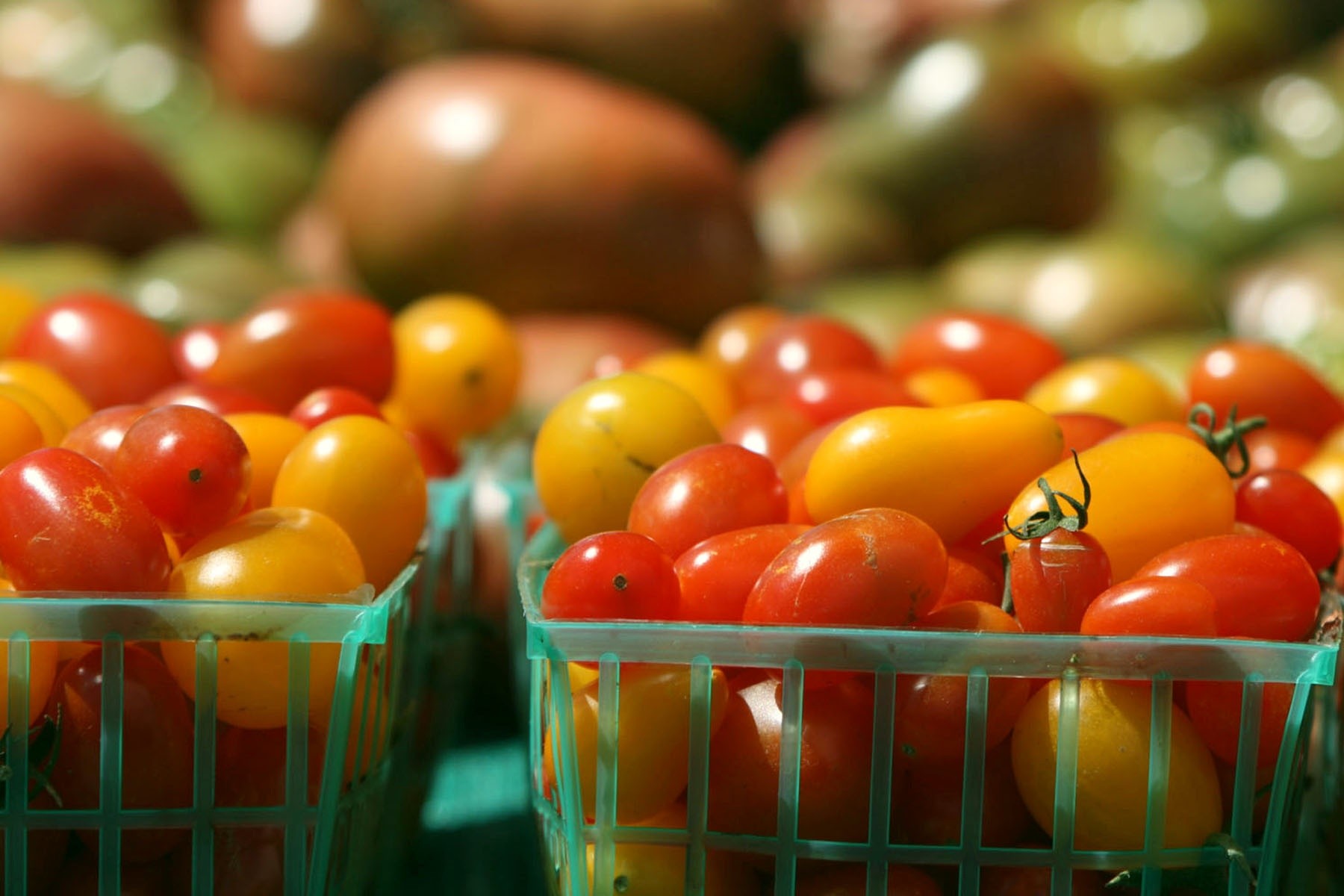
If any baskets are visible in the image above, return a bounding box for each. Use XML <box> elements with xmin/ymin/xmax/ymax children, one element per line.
<box><xmin>0</xmin><ymin>430</ymin><xmax>492</xmax><ymax>896</ymax></box>
<box><xmin>504</xmin><ymin>462</ymin><xmax>1344</xmax><ymax>896</ymax></box>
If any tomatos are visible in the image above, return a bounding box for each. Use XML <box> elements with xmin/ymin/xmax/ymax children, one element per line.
<box><xmin>0</xmin><ymin>1</ymin><xmax>1344</xmax><ymax>883</ymax></box>
